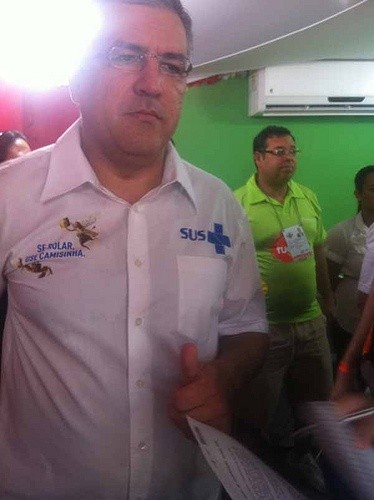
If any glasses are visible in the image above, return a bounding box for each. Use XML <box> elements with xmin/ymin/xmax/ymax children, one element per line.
<box><xmin>260</xmin><ymin>148</ymin><xmax>301</xmax><ymax>157</ymax></box>
<box><xmin>94</xmin><ymin>45</ymin><xmax>193</xmax><ymax>76</ymax></box>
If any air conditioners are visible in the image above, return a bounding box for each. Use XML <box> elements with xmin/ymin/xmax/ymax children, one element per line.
<box><xmin>247</xmin><ymin>60</ymin><xmax>374</xmax><ymax>118</ymax></box>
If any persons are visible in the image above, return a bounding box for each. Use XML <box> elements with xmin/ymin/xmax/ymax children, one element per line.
<box><xmin>0</xmin><ymin>130</ymin><xmax>29</xmax><ymax>163</ymax></box>
<box><xmin>226</xmin><ymin>126</ymin><xmax>340</xmax><ymax>449</ymax></box>
<box><xmin>0</xmin><ymin>0</ymin><xmax>271</xmax><ymax>500</ymax></box>
<box><xmin>320</xmin><ymin>164</ymin><xmax>373</xmax><ymax>401</ymax></box>
<box><xmin>331</xmin><ymin>274</ymin><xmax>374</xmax><ymax>401</ymax></box>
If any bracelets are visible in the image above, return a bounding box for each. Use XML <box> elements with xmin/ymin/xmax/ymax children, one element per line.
<box><xmin>338</xmin><ymin>361</ymin><xmax>353</xmax><ymax>375</ymax></box>
